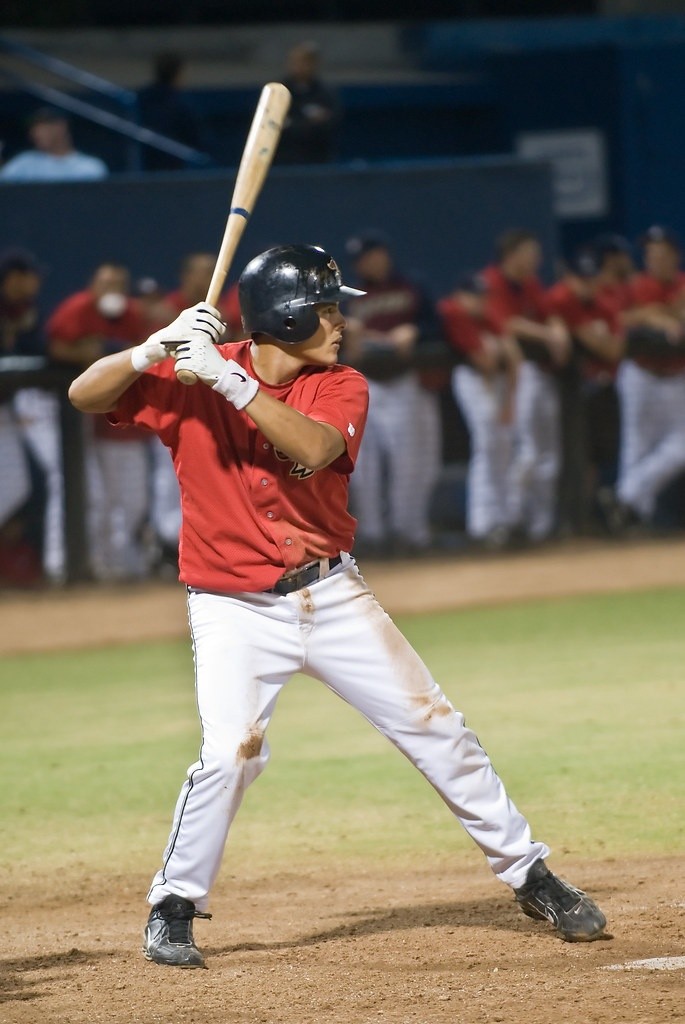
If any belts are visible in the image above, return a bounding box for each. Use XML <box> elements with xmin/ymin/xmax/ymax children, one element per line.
<box><xmin>264</xmin><ymin>557</ymin><xmax>341</xmax><ymax>596</ymax></box>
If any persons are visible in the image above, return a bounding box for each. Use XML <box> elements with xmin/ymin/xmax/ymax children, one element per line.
<box><xmin>136</xmin><ymin>54</ymin><xmax>203</xmax><ymax>170</ymax></box>
<box><xmin>0</xmin><ymin>224</ymin><xmax>685</xmax><ymax>588</ymax></box>
<box><xmin>64</xmin><ymin>240</ymin><xmax>612</xmax><ymax>974</ymax></box>
<box><xmin>273</xmin><ymin>45</ymin><xmax>338</xmax><ymax>163</ymax></box>
<box><xmin>0</xmin><ymin>102</ymin><xmax>111</xmax><ymax>183</ymax></box>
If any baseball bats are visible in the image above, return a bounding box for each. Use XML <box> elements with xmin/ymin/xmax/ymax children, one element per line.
<box><xmin>174</xmin><ymin>79</ymin><xmax>291</xmax><ymax>387</ymax></box>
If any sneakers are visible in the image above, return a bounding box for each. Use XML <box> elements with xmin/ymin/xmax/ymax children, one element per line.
<box><xmin>143</xmin><ymin>894</ymin><xmax>204</xmax><ymax>972</ymax></box>
<box><xmin>517</xmin><ymin>860</ymin><xmax>608</xmax><ymax>943</ymax></box>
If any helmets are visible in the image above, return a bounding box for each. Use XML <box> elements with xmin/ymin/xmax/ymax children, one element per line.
<box><xmin>237</xmin><ymin>243</ymin><xmax>369</xmax><ymax>344</ymax></box>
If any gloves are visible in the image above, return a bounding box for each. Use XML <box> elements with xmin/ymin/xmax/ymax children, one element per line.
<box><xmin>131</xmin><ymin>301</ymin><xmax>260</xmax><ymax>412</ymax></box>
